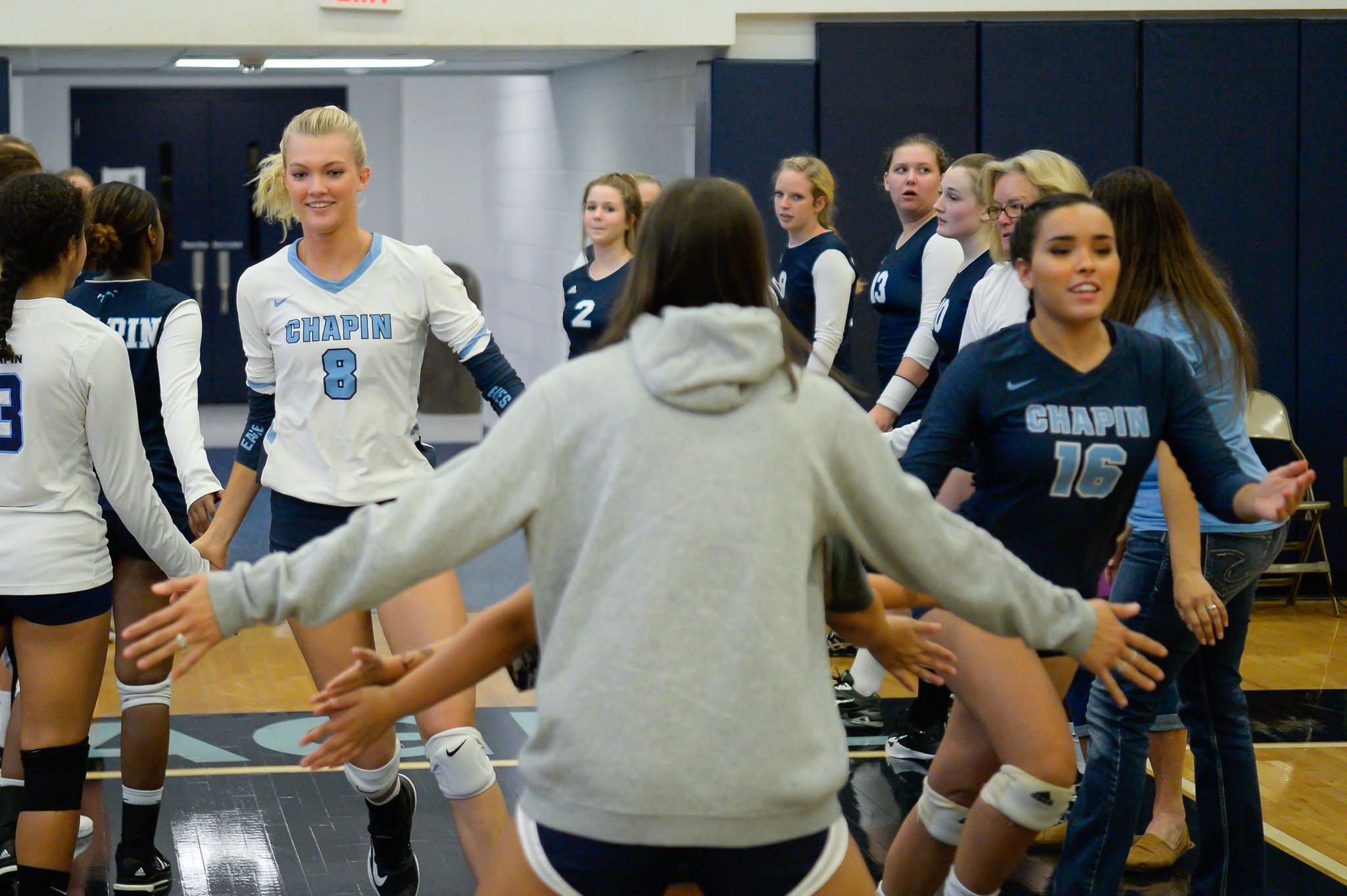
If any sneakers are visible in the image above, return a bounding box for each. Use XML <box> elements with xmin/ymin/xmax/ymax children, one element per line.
<box><xmin>831</xmin><ymin>669</ymin><xmax>907</xmax><ymax>749</ymax></box>
<box><xmin>114</xmin><ymin>844</ymin><xmax>173</xmax><ymax>896</ymax></box>
<box><xmin>365</xmin><ymin>774</ymin><xmax>419</xmax><ymax>896</ymax></box>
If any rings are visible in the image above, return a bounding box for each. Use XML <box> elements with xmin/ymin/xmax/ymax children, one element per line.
<box><xmin>177</xmin><ymin>632</ymin><xmax>189</xmax><ymax>649</ymax></box>
<box><xmin>1127</xmin><ymin>651</ymin><xmax>1139</xmax><ymax>663</ymax></box>
<box><xmin>1118</xmin><ymin>662</ymin><xmax>1126</xmax><ymax>673</ymax></box>
<box><xmin>1206</xmin><ymin>604</ymin><xmax>1218</xmax><ymax>610</ymax></box>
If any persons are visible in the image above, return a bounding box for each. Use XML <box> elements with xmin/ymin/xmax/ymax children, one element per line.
<box><xmin>1</xmin><ymin>135</ymin><xmax>1315</xmax><ymax>896</ymax></box>
<box><xmin>168</xmin><ymin>107</ymin><xmax>526</xmax><ymax>896</ymax></box>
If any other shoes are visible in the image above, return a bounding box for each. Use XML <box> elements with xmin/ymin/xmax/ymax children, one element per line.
<box><xmin>78</xmin><ymin>816</ymin><xmax>94</xmax><ymax>837</ymax></box>
<box><xmin>826</xmin><ymin>630</ymin><xmax>857</xmax><ymax>657</ymax></box>
<box><xmin>0</xmin><ymin>839</ymin><xmax>17</xmax><ymax>874</ymax></box>
<box><xmin>1125</xmin><ymin>826</ymin><xmax>1195</xmax><ymax>879</ymax></box>
<box><xmin>887</xmin><ymin>723</ymin><xmax>945</xmax><ymax>760</ymax></box>
<box><xmin>507</xmin><ymin>646</ymin><xmax>539</xmax><ymax>689</ymax></box>
<box><xmin>1034</xmin><ymin>819</ymin><xmax>1070</xmax><ymax>848</ymax></box>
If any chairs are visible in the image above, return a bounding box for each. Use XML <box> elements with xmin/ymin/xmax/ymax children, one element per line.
<box><xmin>1244</xmin><ymin>387</ymin><xmax>1339</xmax><ymax>617</ymax></box>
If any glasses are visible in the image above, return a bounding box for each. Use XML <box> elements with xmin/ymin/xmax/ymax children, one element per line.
<box><xmin>986</xmin><ymin>204</ymin><xmax>1029</xmax><ymax>221</ymax></box>
